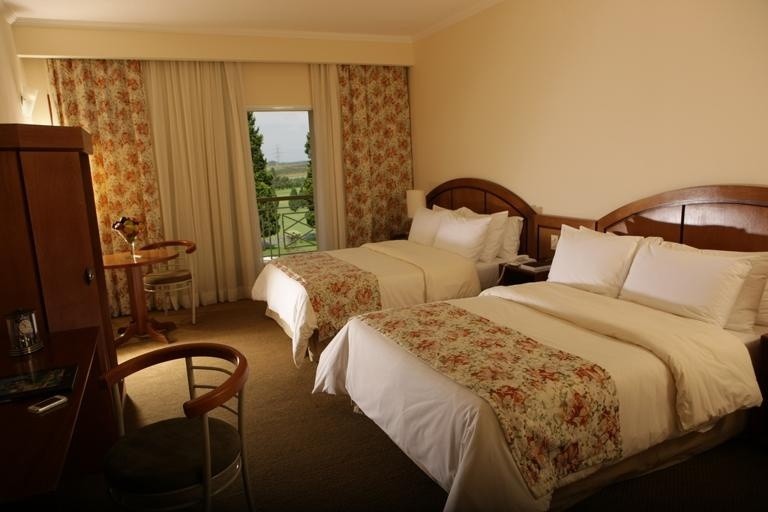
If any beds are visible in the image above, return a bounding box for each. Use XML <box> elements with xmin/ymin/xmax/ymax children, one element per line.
<box><xmin>311</xmin><ymin>184</ymin><xmax>768</xmax><ymax>512</ymax></box>
<box><xmin>251</xmin><ymin>177</ymin><xmax>536</xmax><ymax>369</ymax></box>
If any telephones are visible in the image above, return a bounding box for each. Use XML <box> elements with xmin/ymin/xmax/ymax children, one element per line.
<box><xmin>496</xmin><ymin>254</ymin><xmax>538</xmax><ymax>284</ymax></box>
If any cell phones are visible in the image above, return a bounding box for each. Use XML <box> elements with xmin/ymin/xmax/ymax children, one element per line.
<box><xmin>27</xmin><ymin>394</ymin><xmax>68</xmax><ymax>413</ymax></box>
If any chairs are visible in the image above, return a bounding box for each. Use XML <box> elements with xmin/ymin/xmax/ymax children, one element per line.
<box><xmin>94</xmin><ymin>342</ymin><xmax>252</xmax><ymax>512</ymax></box>
<box><xmin>138</xmin><ymin>239</ymin><xmax>200</xmax><ymax>326</ymax></box>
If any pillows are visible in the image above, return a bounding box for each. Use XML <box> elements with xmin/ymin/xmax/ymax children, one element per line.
<box><xmin>546</xmin><ymin>224</ymin><xmax>643</xmax><ymax>299</ymax></box>
<box><xmin>480</xmin><ymin>214</ymin><xmax>524</xmax><ymax>262</ymax></box>
<box><xmin>452</xmin><ymin>207</ymin><xmax>509</xmax><ymax>264</ymax></box>
<box><xmin>579</xmin><ymin>225</ymin><xmax>663</xmax><ymax>244</ymax></box>
<box><xmin>432</xmin><ymin>211</ymin><xmax>492</xmax><ymax>263</ymax></box>
<box><xmin>407</xmin><ymin>206</ymin><xmax>442</xmax><ymax>246</ymax></box>
<box><xmin>432</xmin><ymin>204</ymin><xmax>451</xmax><ymax>212</ymax></box>
<box><xmin>670</xmin><ymin>243</ymin><xmax>768</xmax><ymax>331</ymax></box>
<box><xmin>606</xmin><ymin>231</ymin><xmax>617</xmax><ymax>237</ymax></box>
<box><xmin>756</xmin><ymin>279</ymin><xmax>768</xmax><ymax>327</ymax></box>
<box><xmin>622</xmin><ymin>242</ymin><xmax>752</xmax><ymax>329</ymax></box>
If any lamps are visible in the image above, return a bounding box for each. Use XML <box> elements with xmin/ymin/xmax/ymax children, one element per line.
<box><xmin>405</xmin><ymin>189</ymin><xmax>427</xmax><ymax>218</ymax></box>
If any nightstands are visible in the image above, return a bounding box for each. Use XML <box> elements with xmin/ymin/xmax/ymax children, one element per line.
<box><xmin>499</xmin><ymin>257</ymin><xmax>551</xmax><ymax>287</ymax></box>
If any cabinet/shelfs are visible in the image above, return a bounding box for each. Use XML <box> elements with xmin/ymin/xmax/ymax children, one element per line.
<box><xmin>0</xmin><ymin>150</ymin><xmax>42</xmax><ymax>332</ymax></box>
<box><xmin>18</xmin><ymin>146</ymin><xmax>104</xmax><ymax>332</ymax></box>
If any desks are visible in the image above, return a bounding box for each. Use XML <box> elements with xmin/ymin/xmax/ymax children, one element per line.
<box><xmin>0</xmin><ymin>325</ymin><xmax>112</xmax><ymax>512</ymax></box>
<box><xmin>101</xmin><ymin>249</ymin><xmax>180</xmax><ymax>348</ymax></box>
